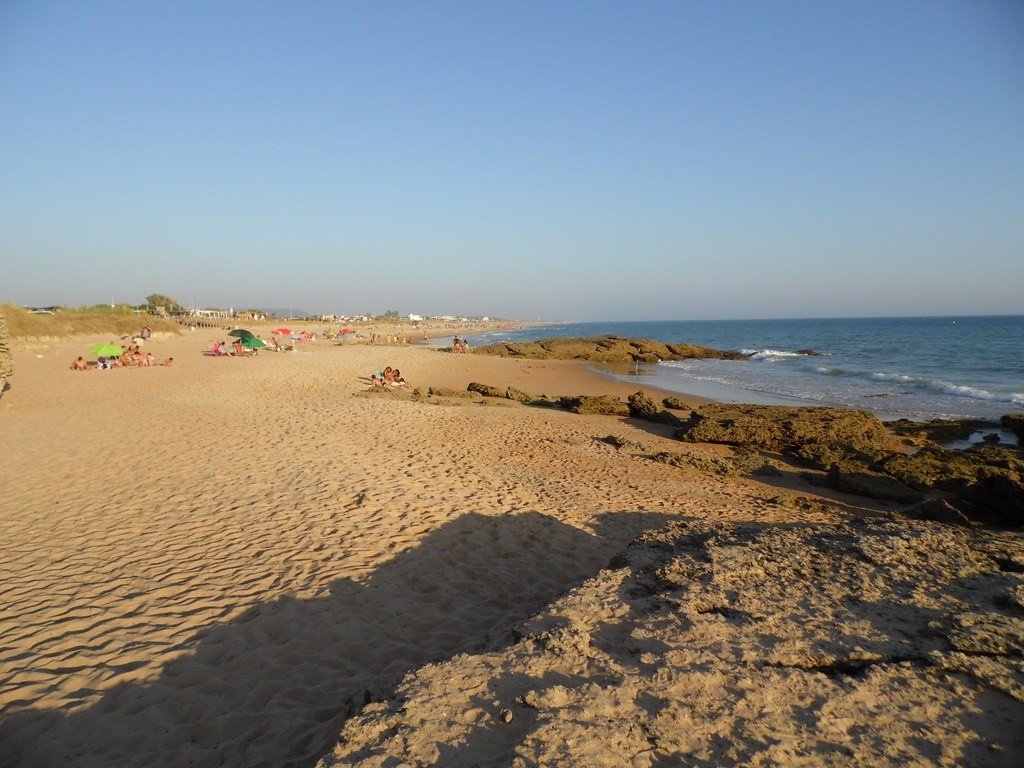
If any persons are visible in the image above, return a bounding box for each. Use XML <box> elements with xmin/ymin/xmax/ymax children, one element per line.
<box><xmin>72</xmin><ymin>356</ymin><xmax>88</xmax><ymax>370</ymax></box>
<box><xmin>213</xmin><ymin>340</ymin><xmax>258</xmax><ymax>357</ymax></box>
<box><xmin>96</xmin><ymin>324</ymin><xmax>173</xmax><ymax>370</ymax></box>
<box><xmin>294</xmin><ymin>319</ymin><xmax>519</xmax><ymax>354</ymax></box>
<box><xmin>371</xmin><ymin>366</ymin><xmax>406</xmax><ymax>390</ymax></box>
<box><xmin>270</xmin><ymin>337</ymin><xmax>282</xmax><ymax>351</ymax></box>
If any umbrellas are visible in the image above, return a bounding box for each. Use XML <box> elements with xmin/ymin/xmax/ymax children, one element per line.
<box><xmin>270</xmin><ymin>327</ymin><xmax>303</xmax><ymax>349</ymax></box>
<box><xmin>228</xmin><ymin>329</ymin><xmax>266</xmax><ymax>356</ymax></box>
<box><xmin>90</xmin><ymin>344</ymin><xmax>124</xmax><ymax>370</ymax></box>
